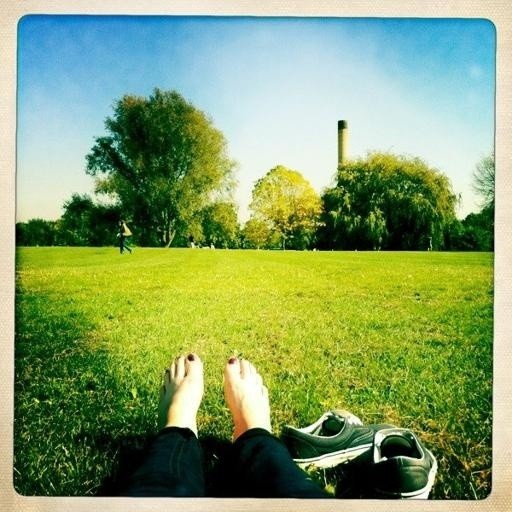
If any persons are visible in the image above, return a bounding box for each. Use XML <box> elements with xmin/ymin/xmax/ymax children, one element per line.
<box><xmin>117</xmin><ymin>220</ymin><xmax>133</xmax><ymax>254</ymax></box>
<box><xmin>92</xmin><ymin>353</ymin><xmax>336</xmax><ymax>499</ymax></box>
<box><xmin>188</xmin><ymin>234</ymin><xmax>216</xmax><ymax>249</ymax></box>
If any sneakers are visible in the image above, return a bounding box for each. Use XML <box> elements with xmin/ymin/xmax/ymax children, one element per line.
<box><xmin>368</xmin><ymin>428</ymin><xmax>439</xmax><ymax>500</ymax></box>
<box><xmin>279</xmin><ymin>408</ymin><xmax>398</xmax><ymax>473</ymax></box>
